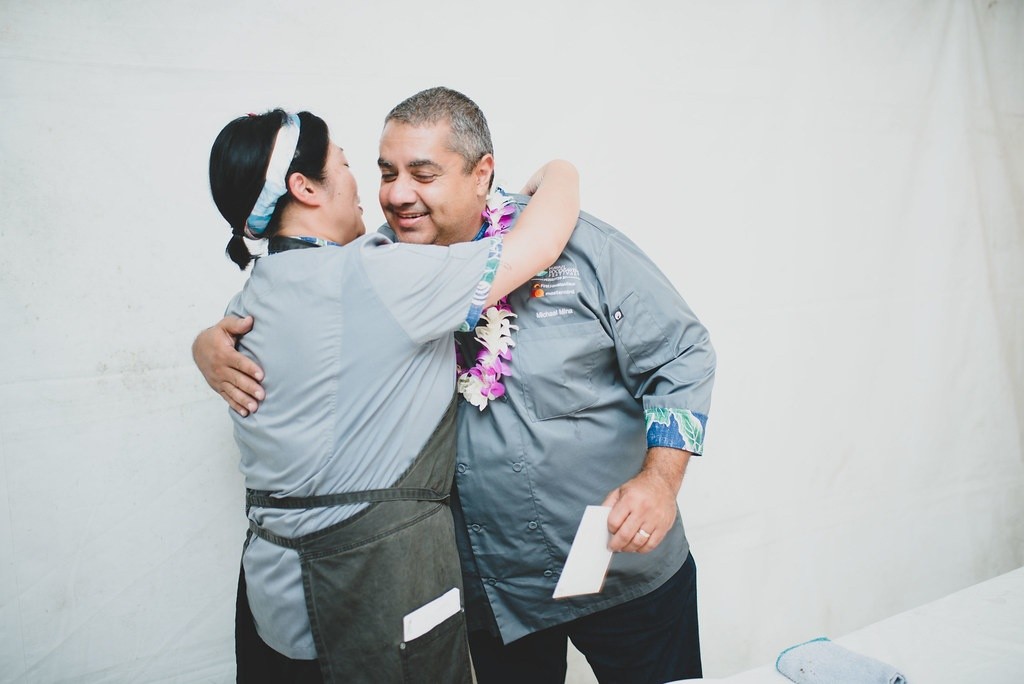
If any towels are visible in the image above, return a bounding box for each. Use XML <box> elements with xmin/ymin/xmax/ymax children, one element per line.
<box><xmin>774</xmin><ymin>636</ymin><xmax>908</xmax><ymax>684</ymax></box>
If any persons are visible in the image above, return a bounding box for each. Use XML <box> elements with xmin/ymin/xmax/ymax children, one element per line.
<box><xmin>203</xmin><ymin>104</ymin><xmax>584</xmax><ymax>682</ymax></box>
<box><xmin>189</xmin><ymin>85</ymin><xmax>720</xmax><ymax>684</ymax></box>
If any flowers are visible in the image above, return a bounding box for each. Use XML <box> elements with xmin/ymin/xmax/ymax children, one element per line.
<box><xmin>451</xmin><ymin>191</ymin><xmax>525</xmax><ymax>414</ymax></box>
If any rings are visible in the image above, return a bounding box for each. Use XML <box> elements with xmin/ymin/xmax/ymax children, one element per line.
<box><xmin>639</xmin><ymin>529</ymin><xmax>651</xmax><ymax>538</ymax></box>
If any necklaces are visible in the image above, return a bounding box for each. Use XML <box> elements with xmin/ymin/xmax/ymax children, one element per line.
<box><xmin>416</xmin><ymin>188</ymin><xmax>524</xmax><ymax>409</ymax></box>
